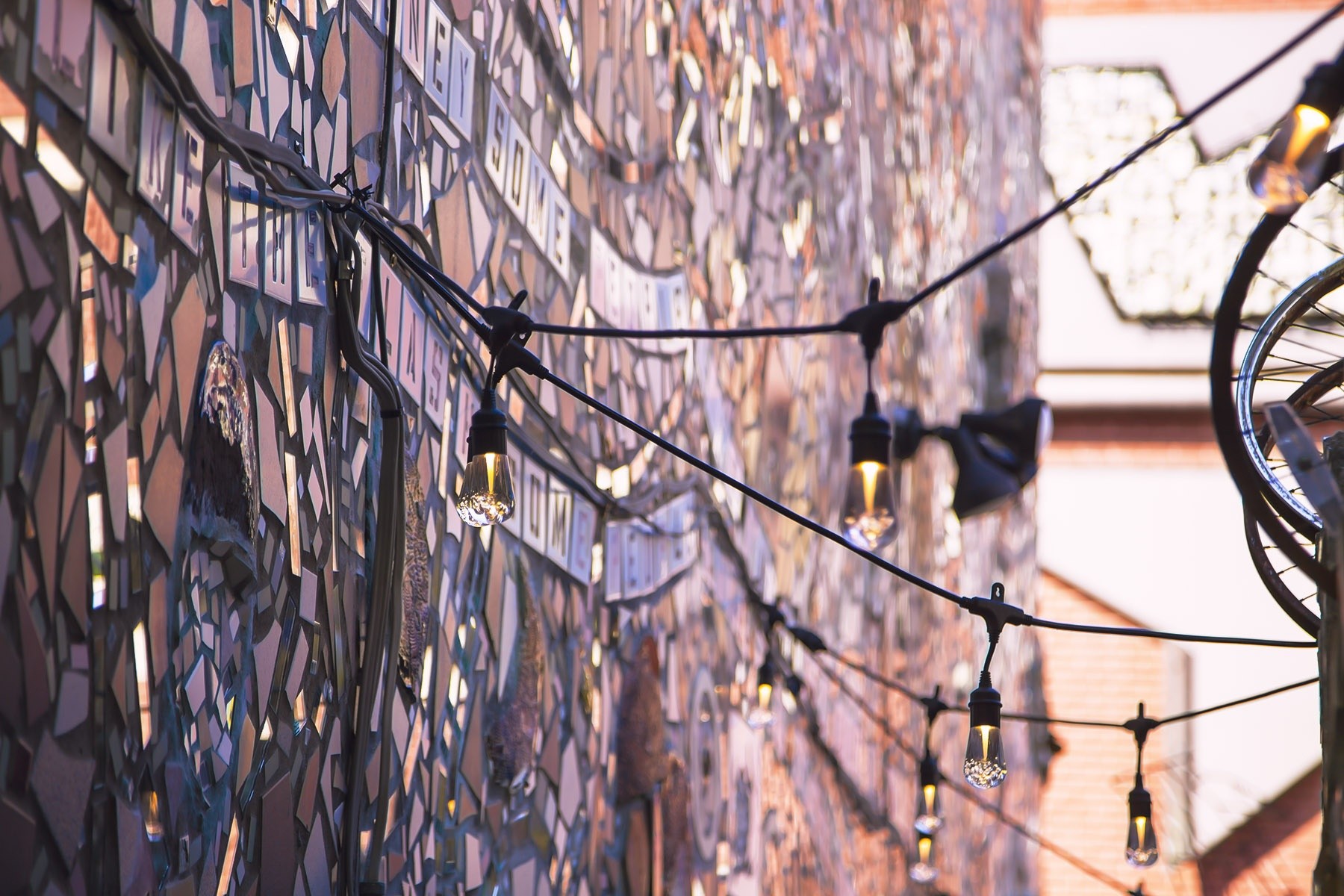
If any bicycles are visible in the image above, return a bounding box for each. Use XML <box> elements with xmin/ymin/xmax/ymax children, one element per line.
<box><xmin>1204</xmin><ymin>140</ymin><xmax>1344</xmax><ymax>642</ymax></box>
